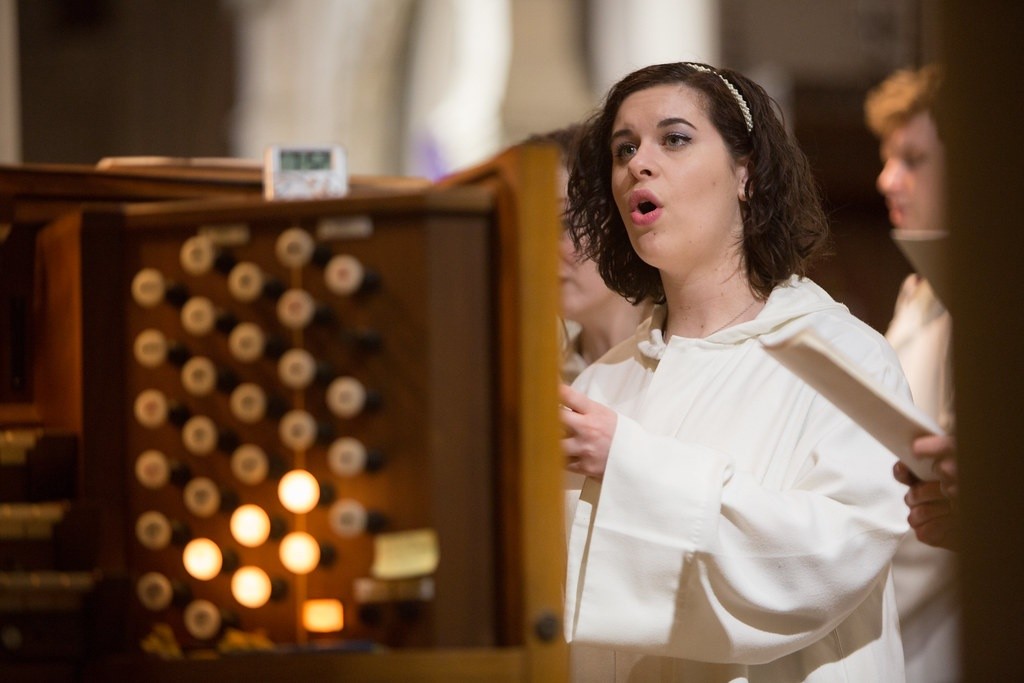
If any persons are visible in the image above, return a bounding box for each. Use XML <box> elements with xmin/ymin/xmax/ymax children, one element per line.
<box><xmin>1</xmin><ymin>191</ymin><xmax>37</xmax><ymax>405</ymax></box>
<box><xmin>528</xmin><ymin>37</ymin><xmax>1024</xmax><ymax>683</ymax></box>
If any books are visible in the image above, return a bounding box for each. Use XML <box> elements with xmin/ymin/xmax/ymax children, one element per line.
<box><xmin>761</xmin><ymin>326</ymin><xmax>950</xmax><ymax>482</ymax></box>
